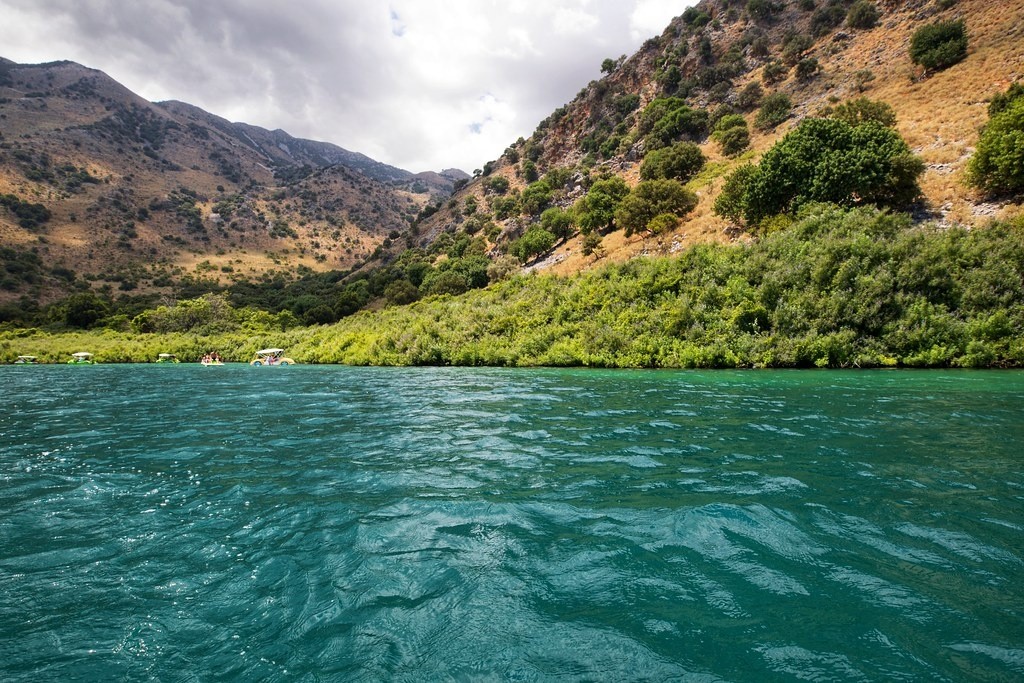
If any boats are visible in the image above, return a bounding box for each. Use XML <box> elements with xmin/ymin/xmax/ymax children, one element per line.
<box><xmin>201</xmin><ymin>358</ymin><xmax>225</xmax><ymax>367</ymax></box>
<box><xmin>14</xmin><ymin>355</ymin><xmax>38</xmax><ymax>365</ymax></box>
<box><xmin>68</xmin><ymin>353</ymin><xmax>97</xmax><ymax>365</ymax></box>
<box><xmin>249</xmin><ymin>349</ymin><xmax>295</xmax><ymax>366</ymax></box>
<box><xmin>156</xmin><ymin>354</ymin><xmax>181</xmax><ymax>363</ymax></box>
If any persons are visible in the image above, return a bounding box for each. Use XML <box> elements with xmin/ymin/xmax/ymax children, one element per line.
<box><xmin>204</xmin><ymin>350</ymin><xmax>217</xmax><ymax>364</ymax></box>
<box><xmin>265</xmin><ymin>353</ymin><xmax>276</xmax><ymax>365</ymax></box>
